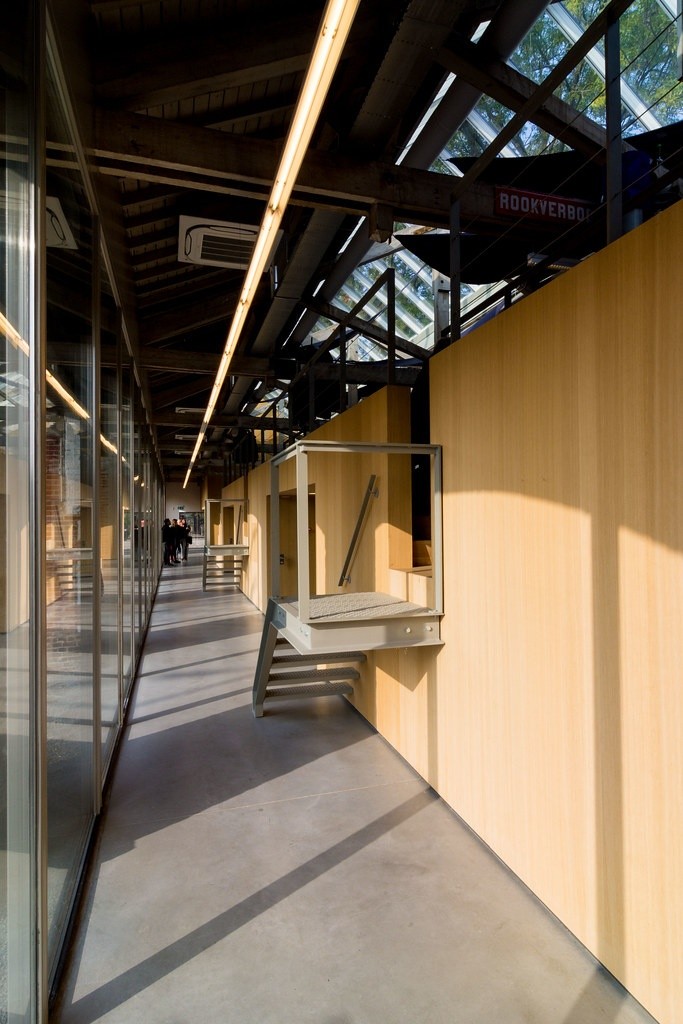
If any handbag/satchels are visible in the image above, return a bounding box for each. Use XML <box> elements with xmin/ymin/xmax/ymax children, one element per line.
<box><xmin>187</xmin><ymin>536</ymin><xmax>192</xmax><ymax>544</ymax></box>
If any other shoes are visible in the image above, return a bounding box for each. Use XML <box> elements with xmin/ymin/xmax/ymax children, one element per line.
<box><xmin>171</xmin><ymin>560</ymin><xmax>181</xmax><ymax>563</ymax></box>
<box><xmin>164</xmin><ymin>563</ymin><xmax>173</xmax><ymax>567</ymax></box>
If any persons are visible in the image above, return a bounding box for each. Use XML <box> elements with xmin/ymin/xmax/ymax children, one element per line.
<box><xmin>134</xmin><ymin>518</ymin><xmax>154</xmax><ymax>565</ymax></box>
<box><xmin>161</xmin><ymin>518</ymin><xmax>192</xmax><ymax>568</ymax></box>
